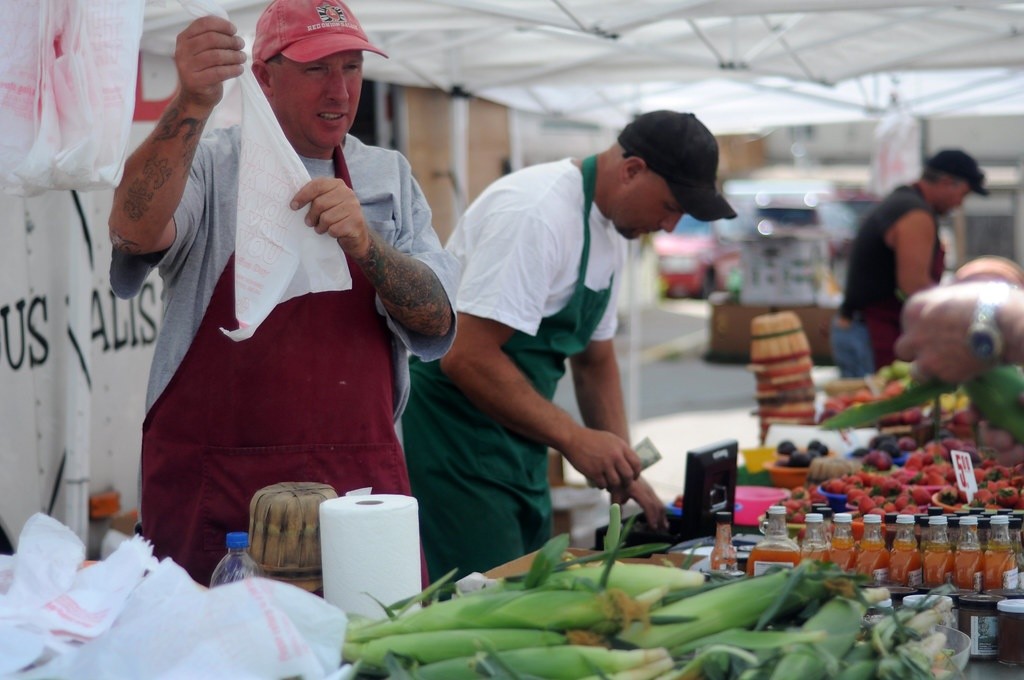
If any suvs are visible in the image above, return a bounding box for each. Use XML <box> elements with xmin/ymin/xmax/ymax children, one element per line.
<box><xmin>650</xmin><ymin>169</ymin><xmax>885</xmax><ymax>301</ymax></box>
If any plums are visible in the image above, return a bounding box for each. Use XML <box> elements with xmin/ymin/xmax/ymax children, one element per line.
<box><xmin>778</xmin><ymin>436</ymin><xmax>903</xmax><ymax>470</ymax></box>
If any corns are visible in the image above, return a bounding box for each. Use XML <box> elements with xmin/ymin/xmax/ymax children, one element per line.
<box><xmin>337</xmin><ymin>564</ymin><xmax>952</xmax><ymax>680</ymax></box>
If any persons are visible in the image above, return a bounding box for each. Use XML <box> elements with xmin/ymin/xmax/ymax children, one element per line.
<box><xmin>835</xmin><ymin>148</ymin><xmax>990</xmax><ymax>380</ymax></box>
<box><xmin>392</xmin><ymin>109</ymin><xmax>738</xmax><ymax>595</ymax></box>
<box><xmin>107</xmin><ymin>0</ymin><xmax>457</xmax><ymax>596</ymax></box>
<box><xmin>898</xmin><ymin>256</ymin><xmax>1024</xmax><ymax>384</ymax></box>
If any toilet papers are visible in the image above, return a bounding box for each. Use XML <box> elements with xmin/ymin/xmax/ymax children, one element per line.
<box><xmin>318</xmin><ymin>486</ymin><xmax>421</xmax><ymax>625</ymax></box>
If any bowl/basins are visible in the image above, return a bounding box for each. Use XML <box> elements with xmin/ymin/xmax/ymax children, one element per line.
<box><xmin>734</xmin><ymin>485</ymin><xmax>791</xmax><ymax>525</ymax></box>
<box><xmin>817</xmin><ymin>483</ymin><xmax>848</xmax><ymax>511</ymax></box>
<box><xmin>742</xmin><ymin>447</ymin><xmax>781</xmax><ymax>473</ymax></box>
<box><xmin>934</xmin><ymin>625</ymin><xmax>972</xmax><ymax>679</ymax></box>
<box><xmin>766</xmin><ymin>466</ymin><xmax>810</xmax><ymax>488</ymax></box>
<box><xmin>931</xmin><ymin>492</ymin><xmax>958</xmax><ymax>512</ymax></box>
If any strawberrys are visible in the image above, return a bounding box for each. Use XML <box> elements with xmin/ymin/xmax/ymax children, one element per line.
<box><xmin>775</xmin><ymin>451</ymin><xmax>1023</xmax><ymax>516</ymax></box>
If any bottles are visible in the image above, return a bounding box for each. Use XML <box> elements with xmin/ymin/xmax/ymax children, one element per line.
<box><xmin>209</xmin><ymin>532</ymin><xmax>265</xmax><ymax>590</ymax></box>
<box><xmin>855</xmin><ymin>507</ymin><xmax>1024</xmax><ymax>667</ymax></box>
<box><xmin>88</xmin><ymin>491</ymin><xmax>121</xmax><ymax>563</ymax></box>
<box><xmin>710</xmin><ymin>512</ymin><xmax>737</xmax><ymax>571</ymax></box>
<box><xmin>100</xmin><ymin>512</ymin><xmax>136</xmax><ymax>560</ymax></box>
<box><xmin>811</xmin><ymin>503</ymin><xmax>827</xmax><ymax>513</ymax></box>
<box><xmin>816</xmin><ymin>507</ymin><xmax>833</xmax><ymax>542</ymax></box>
<box><xmin>830</xmin><ymin>513</ymin><xmax>856</xmax><ymax>573</ymax></box>
<box><xmin>797</xmin><ymin>513</ymin><xmax>830</xmax><ymax>566</ymax></box>
<box><xmin>747</xmin><ymin>505</ymin><xmax>801</xmax><ymax>577</ymax></box>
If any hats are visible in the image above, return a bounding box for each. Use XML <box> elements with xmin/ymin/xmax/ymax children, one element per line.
<box><xmin>252</xmin><ymin>0</ymin><xmax>391</xmax><ymax>64</ymax></box>
<box><xmin>930</xmin><ymin>150</ymin><xmax>987</xmax><ymax>195</ymax></box>
<box><xmin>618</xmin><ymin>111</ymin><xmax>739</xmax><ymax>221</ymax></box>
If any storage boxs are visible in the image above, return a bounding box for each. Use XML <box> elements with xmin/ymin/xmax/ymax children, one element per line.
<box><xmin>594</xmin><ymin>438</ymin><xmax>738</xmax><ymax>552</ymax></box>
<box><xmin>482</xmin><ymin>542</ymin><xmax>705</xmax><ymax>583</ymax></box>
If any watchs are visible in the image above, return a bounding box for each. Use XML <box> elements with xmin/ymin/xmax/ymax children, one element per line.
<box><xmin>967</xmin><ymin>283</ymin><xmax>1004</xmax><ymax>372</ymax></box>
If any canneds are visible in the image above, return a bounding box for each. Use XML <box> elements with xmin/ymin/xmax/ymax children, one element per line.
<box><xmin>857</xmin><ymin>593</ymin><xmax>1024</xmax><ymax>668</ymax></box>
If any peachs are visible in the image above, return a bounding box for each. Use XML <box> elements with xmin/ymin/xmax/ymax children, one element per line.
<box><xmin>862</xmin><ymin>406</ymin><xmax>980</xmax><ymax>470</ymax></box>
